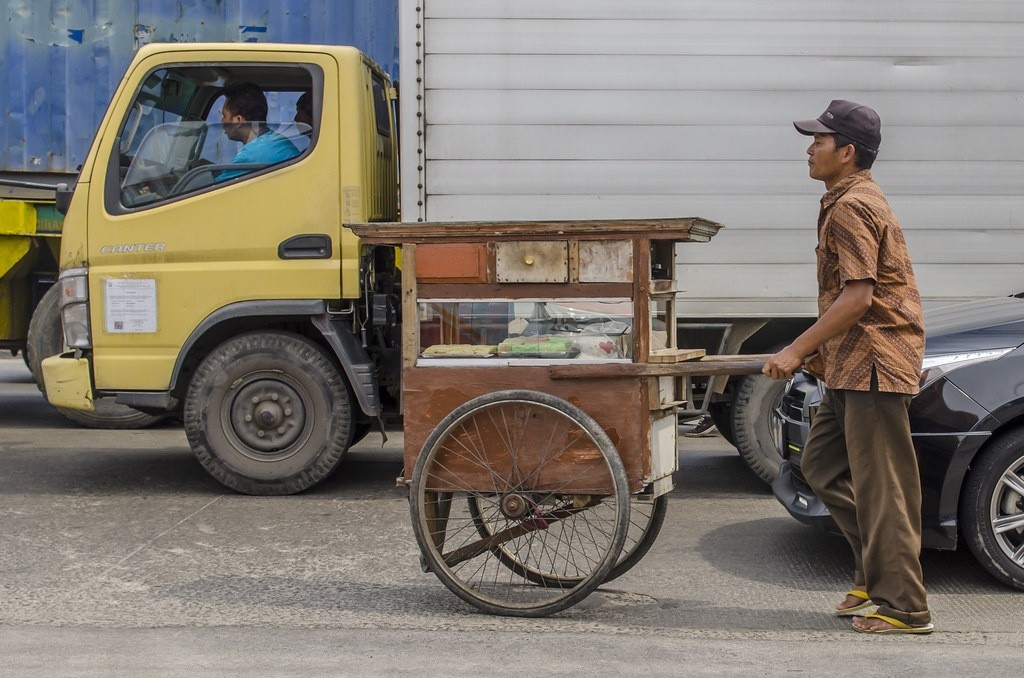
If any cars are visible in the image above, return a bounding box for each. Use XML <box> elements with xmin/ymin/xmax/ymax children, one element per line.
<box><xmin>769</xmin><ymin>286</ymin><xmax>1024</xmax><ymax>594</ymax></box>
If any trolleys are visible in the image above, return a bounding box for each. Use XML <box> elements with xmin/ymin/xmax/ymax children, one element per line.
<box><xmin>341</xmin><ymin>215</ymin><xmax>819</xmax><ymax>620</ymax></box>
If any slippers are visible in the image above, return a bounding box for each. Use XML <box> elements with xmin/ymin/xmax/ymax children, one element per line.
<box><xmin>835</xmin><ymin>590</ymin><xmax>877</xmax><ymax>616</ymax></box>
<box><xmin>852</xmin><ymin>610</ymin><xmax>934</xmax><ymax>634</ymax></box>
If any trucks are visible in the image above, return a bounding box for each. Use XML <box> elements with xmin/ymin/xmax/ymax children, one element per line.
<box><xmin>42</xmin><ymin>2</ymin><xmax>1024</xmax><ymax>498</ymax></box>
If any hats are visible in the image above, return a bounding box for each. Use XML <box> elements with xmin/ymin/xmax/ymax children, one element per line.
<box><xmin>794</xmin><ymin>100</ymin><xmax>882</xmax><ymax>150</ymax></box>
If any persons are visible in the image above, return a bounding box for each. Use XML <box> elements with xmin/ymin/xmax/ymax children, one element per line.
<box><xmin>294</xmin><ymin>92</ymin><xmax>314</xmax><ymax>154</ymax></box>
<box><xmin>761</xmin><ymin>99</ymin><xmax>935</xmax><ymax>634</ymax></box>
<box><xmin>139</xmin><ymin>83</ymin><xmax>301</xmax><ymax>200</ymax></box>
